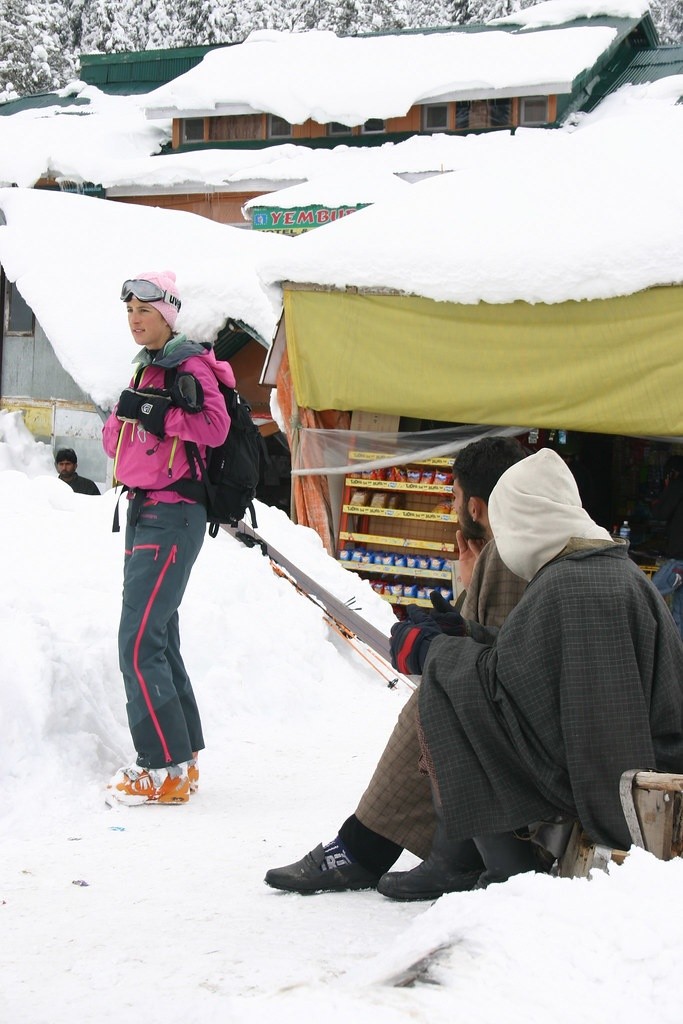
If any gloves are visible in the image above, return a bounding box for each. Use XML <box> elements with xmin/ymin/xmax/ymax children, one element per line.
<box><xmin>428</xmin><ymin>591</ymin><xmax>467</xmax><ymax>636</ymax></box>
<box><xmin>115</xmin><ymin>389</ymin><xmax>172</xmax><ymax>441</ymax></box>
<box><xmin>389</xmin><ymin>604</ymin><xmax>443</xmax><ymax>676</ymax></box>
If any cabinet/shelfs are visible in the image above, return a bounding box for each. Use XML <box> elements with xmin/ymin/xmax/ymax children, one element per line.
<box><xmin>334</xmin><ymin>435</ymin><xmax>458</xmax><ymax>608</ymax></box>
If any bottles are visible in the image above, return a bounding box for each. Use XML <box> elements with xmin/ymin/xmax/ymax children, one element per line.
<box><xmin>620</xmin><ymin>521</ymin><xmax>630</xmax><ymax>539</ymax></box>
<box><xmin>612</xmin><ymin>525</ymin><xmax>619</xmax><ymax>535</ymax></box>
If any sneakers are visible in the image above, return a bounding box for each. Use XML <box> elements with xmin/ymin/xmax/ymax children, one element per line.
<box><xmin>104</xmin><ymin>762</ymin><xmax>190</xmax><ymax>806</ymax></box>
<box><xmin>108</xmin><ymin>763</ymin><xmax>199</xmax><ymax>795</ymax></box>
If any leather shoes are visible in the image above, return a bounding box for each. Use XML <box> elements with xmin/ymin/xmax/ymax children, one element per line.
<box><xmin>376</xmin><ymin>853</ymin><xmax>487</xmax><ymax>901</ymax></box>
<box><xmin>263</xmin><ymin>842</ymin><xmax>379</xmax><ymax>894</ymax></box>
<box><xmin>472</xmin><ymin>864</ymin><xmax>543</xmax><ymax>890</ymax></box>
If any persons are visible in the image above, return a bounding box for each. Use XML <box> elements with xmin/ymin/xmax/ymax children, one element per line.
<box><xmin>100</xmin><ymin>271</ymin><xmax>236</xmax><ymax>806</ymax></box>
<box><xmin>377</xmin><ymin>447</ymin><xmax>683</xmax><ymax>901</ymax></box>
<box><xmin>265</xmin><ymin>436</ymin><xmax>536</xmax><ymax>896</ymax></box>
<box><xmin>652</xmin><ymin>454</ymin><xmax>683</xmax><ymax>643</ymax></box>
<box><xmin>55</xmin><ymin>448</ymin><xmax>99</xmax><ymax>496</ymax></box>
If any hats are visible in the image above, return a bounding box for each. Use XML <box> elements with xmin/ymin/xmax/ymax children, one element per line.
<box><xmin>134</xmin><ymin>270</ymin><xmax>180</xmax><ymax>330</ymax></box>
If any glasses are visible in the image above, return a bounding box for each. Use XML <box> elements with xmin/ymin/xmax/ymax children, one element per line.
<box><xmin>120</xmin><ymin>279</ymin><xmax>181</xmax><ymax>312</ymax></box>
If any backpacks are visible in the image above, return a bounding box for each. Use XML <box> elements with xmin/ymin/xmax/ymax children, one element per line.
<box><xmin>112</xmin><ymin>365</ymin><xmax>271</xmax><ymax>532</ymax></box>
